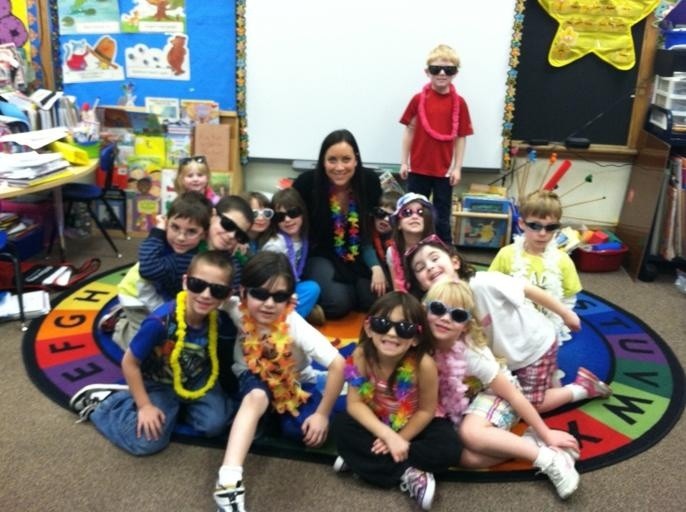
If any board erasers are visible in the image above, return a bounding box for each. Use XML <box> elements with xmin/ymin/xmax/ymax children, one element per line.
<box><xmin>529</xmin><ymin>139</ymin><xmax>548</xmax><ymax>145</ymax></box>
<box><xmin>565</xmin><ymin>138</ymin><xmax>590</xmax><ymax>149</ymax></box>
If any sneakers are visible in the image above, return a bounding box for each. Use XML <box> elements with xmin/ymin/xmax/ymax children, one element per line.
<box><xmin>98</xmin><ymin>304</ymin><xmax>127</xmax><ymax>334</ymax></box>
<box><xmin>576</xmin><ymin>364</ymin><xmax>613</xmax><ymax>399</ymax></box>
<box><xmin>332</xmin><ymin>453</ymin><xmax>353</xmax><ymax>475</ymax></box>
<box><xmin>305</xmin><ymin>303</ymin><xmax>328</xmax><ymax>325</ymax></box>
<box><xmin>521</xmin><ymin>424</ymin><xmax>581</xmax><ymax>502</ymax></box>
<box><xmin>67</xmin><ymin>382</ymin><xmax>130</xmax><ymax>421</ymax></box>
<box><xmin>398</xmin><ymin>465</ymin><xmax>437</xmax><ymax>512</ymax></box>
<box><xmin>213</xmin><ymin>476</ymin><xmax>248</xmax><ymax>512</ymax></box>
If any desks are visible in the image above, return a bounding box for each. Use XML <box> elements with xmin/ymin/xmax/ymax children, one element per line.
<box><xmin>0</xmin><ymin>141</ymin><xmax>100</xmax><ymax>267</ymax></box>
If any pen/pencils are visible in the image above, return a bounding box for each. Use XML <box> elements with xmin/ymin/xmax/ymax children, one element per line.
<box><xmin>91</xmin><ymin>98</ymin><xmax>100</xmax><ymax>111</ymax></box>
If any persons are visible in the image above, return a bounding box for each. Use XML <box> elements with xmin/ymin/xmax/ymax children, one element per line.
<box><xmin>398</xmin><ymin>43</ymin><xmax>474</xmax><ymax>245</ymax></box>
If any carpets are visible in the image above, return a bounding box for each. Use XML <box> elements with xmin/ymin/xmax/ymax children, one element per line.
<box><xmin>20</xmin><ymin>258</ymin><xmax>686</xmax><ymax>486</ymax></box>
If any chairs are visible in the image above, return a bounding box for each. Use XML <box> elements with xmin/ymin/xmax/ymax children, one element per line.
<box><xmin>41</xmin><ymin>141</ymin><xmax>135</xmax><ymax>263</ymax></box>
<box><xmin>0</xmin><ymin>220</ymin><xmax>38</xmax><ymax>333</ymax></box>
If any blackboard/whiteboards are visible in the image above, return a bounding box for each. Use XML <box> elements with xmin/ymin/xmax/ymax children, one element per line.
<box><xmin>500</xmin><ymin>0</ymin><xmax>662</xmax><ymax>157</ymax></box>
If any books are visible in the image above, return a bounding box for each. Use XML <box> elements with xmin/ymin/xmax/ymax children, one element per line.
<box><xmin>68</xmin><ymin>94</ymin><xmax>220</xmax><ymax>128</ymax></box>
<box><xmin>0</xmin><ymin>41</ymin><xmax>68</xmax><ymax>323</ymax></box>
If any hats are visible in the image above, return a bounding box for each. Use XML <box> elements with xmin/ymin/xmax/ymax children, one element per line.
<box><xmin>389</xmin><ymin>191</ymin><xmax>430</xmax><ymax>230</ymax></box>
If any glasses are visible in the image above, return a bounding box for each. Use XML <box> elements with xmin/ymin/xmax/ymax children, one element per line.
<box><xmin>427</xmin><ymin>298</ymin><xmax>473</xmax><ymax>323</ymax></box>
<box><xmin>401</xmin><ymin>233</ymin><xmax>452</xmax><ymax>259</ymax></box>
<box><xmin>252</xmin><ymin>207</ymin><xmax>275</xmax><ymax>220</ymax></box>
<box><xmin>427</xmin><ymin>64</ymin><xmax>458</xmax><ymax>76</ymax></box>
<box><xmin>216</xmin><ymin>211</ymin><xmax>250</xmax><ymax>245</ymax></box>
<box><xmin>372</xmin><ymin>206</ymin><xmax>394</xmax><ymax>220</ymax></box>
<box><xmin>178</xmin><ymin>155</ymin><xmax>210</xmax><ymax>175</ymax></box>
<box><xmin>273</xmin><ymin>206</ymin><xmax>304</xmax><ymax>224</ymax></box>
<box><xmin>168</xmin><ymin>219</ymin><xmax>208</xmax><ymax>238</ymax></box>
<box><xmin>247</xmin><ymin>287</ymin><xmax>293</xmax><ymax>303</ymax></box>
<box><xmin>522</xmin><ymin>220</ymin><xmax>560</xmax><ymax>232</ymax></box>
<box><xmin>186</xmin><ymin>275</ymin><xmax>232</xmax><ymax>300</ymax></box>
<box><xmin>367</xmin><ymin>315</ymin><xmax>418</xmax><ymax>339</ymax></box>
<box><xmin>397</xmin><ymin>206</ymin><xmax>425</xmax><ymax>219</ymax></box>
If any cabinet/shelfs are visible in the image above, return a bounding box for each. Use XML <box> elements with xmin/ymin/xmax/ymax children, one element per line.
<box><xmin>635</xmin><ymin>102</ymin><xmax>686</xmax><ymax>282</ymax></box>
<box><xmin>647</xmin><ymin>71</ymin><xmax>686</xmax><ymax>134</ymax></box>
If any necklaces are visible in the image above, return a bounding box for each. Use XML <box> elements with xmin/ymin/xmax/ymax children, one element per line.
<box><xmin>418</xmin><ymin>81</ymin><xmax>459</xmax><ymax>142</ymax></box>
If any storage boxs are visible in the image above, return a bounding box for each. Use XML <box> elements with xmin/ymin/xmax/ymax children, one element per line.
<box><xmin>573</xmin><ymin>233</ymin><xmax>629</xmax><ymax>274</ymax></box>
